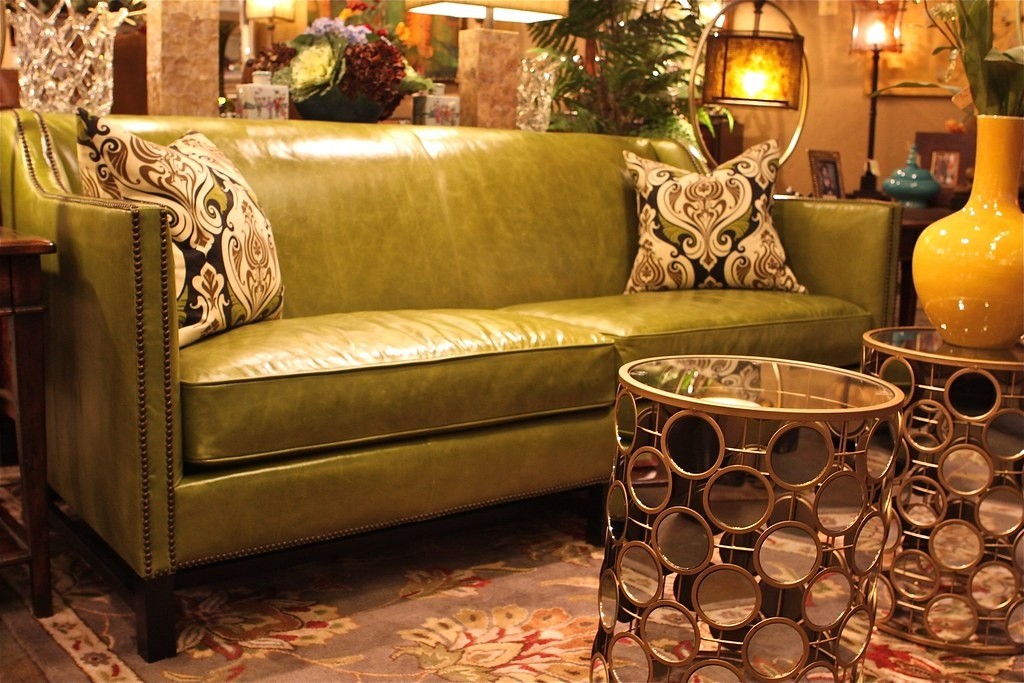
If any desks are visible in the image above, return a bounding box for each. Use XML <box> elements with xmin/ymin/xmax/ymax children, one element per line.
<box><xmin>589</xmin><ymin>355</ymin><xmax>904</xmax><ymax>683</ymax></box>
<box><xmin>861</xmin><ymin>326</ymin><xmax>1023</xmax><ymax>657</ymax></box>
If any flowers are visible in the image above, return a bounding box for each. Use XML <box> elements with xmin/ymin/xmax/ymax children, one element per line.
<box><xmin>269</xmin><ymin>0</ymin><xmax>433</xmax><ymax>122</ymax></box>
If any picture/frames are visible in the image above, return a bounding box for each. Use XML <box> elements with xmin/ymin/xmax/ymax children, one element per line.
<box><xmin>808</xmin><ymin>149</ymin><xmax>845</xmax><ymax>200</ymax></box>
<box><xmin>916</xmin><ymin>132</ymin><xmax>978</xmax><ymax>200</ymax></box>
<box><xmin>863</xmin><ymin>0</ymin><xmax>1024</xmax><ymax>96</ymax></box>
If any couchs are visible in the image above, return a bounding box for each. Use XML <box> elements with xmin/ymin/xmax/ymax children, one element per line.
<box><xmin>0</xmin><ymin>108</ymin><xmax>902</xmax><ymax>664</ymax></box>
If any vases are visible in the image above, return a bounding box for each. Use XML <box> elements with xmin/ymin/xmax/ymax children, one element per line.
<box><xmin>294</xmin><ymin>86</ymin><xmax>387</xmax><ymax>122</ymax></box>
<box><xmin>911</xmin><ymin>114</ymin><xmax>1023</xmax><ymax>347</ymax></box>
<box><xmin>881</xmin><ymin>145</ymin><xmax>941</xmax><ymax>208</ymax></box>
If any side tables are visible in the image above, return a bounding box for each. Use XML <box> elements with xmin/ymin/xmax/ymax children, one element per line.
<box><xmin>0</xmin><ymin>224</ymin><xmax>58</xmax><ymax>619</ymax></box>
<box><xmin>900</xmin><ymin>205</ymin><xmax>967</xmax><ymax>326</ymax></box>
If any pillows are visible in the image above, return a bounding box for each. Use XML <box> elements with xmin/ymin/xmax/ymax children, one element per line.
<box><xmin>623</xmin><ymin>139</ymin><xmax>808</xmax><ymax>295</ymax></box>
<box><xmin>74</xmin><ymin>106</ymin><xmax>284</xmax><ymax>348</ymax></box>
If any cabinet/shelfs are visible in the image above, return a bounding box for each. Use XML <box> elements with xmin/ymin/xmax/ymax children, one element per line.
<box><xmin>698</xmin><ymin>116</ymin><xmax>744</xmax><ymax>164</ymax></box>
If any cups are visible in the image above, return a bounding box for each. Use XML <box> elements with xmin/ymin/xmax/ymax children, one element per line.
<box><xmin>513</xmin><ymin>59</ymin><xmax>554</xmax><ymax>132</ymax></box>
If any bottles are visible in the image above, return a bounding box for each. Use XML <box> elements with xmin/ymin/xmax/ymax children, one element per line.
<box><xmin>411</xmin><ymin>82</ymin><xmax>464</xmax><ymax>125</ymax></box>
<box><xmin>911</xmin><ymin>114</ymin><xmax>1024</xmax><ymax>348</ymax></box>
<box><xmin>234</xmin><ymin>69</ymin><xmax>290</xmax><ymax>122</ymax></box>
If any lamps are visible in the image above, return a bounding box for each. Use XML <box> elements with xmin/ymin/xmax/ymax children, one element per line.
<box><xmin>403</xmin><ymin>0</ymin><xmax>570</xmax><ymax>130</ymax></box>
<box><xmin>688</xmin><ymin>0</ymin><xmax>810</xmax><ymax>170</ymax></box>
<box><xmin>244</xmin><ymin>0</ymin><xmax>296</xmax><ymax>48</ymax></box>
<box><xmin>849</xmin><ymin>0</ymin><xmax>907</xmax><ymax>201</ymax></box>
